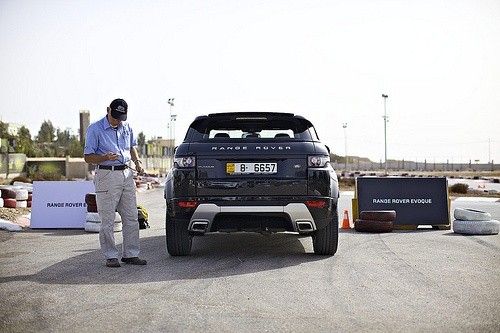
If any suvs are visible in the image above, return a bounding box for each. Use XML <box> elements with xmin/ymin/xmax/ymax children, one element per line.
<box><xmin>163</xmin><ymin>112</ymin><xmax>340</xmax><ymax>256</ymax></box>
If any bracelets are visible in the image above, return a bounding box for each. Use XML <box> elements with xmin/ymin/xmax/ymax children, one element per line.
<box><xmin>133</xmin><ymin>159</ymin><xmax>142</xmax><ymax>165</ymax></box>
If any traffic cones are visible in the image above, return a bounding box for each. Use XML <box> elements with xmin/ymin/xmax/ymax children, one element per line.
<box><xmin>341</xmin><ymin>210</ymin><xmax>351</xmax><ymax>229</ymax></box>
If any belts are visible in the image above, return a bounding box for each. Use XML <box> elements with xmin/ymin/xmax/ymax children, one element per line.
<box><xmin>96</xmin><ymin>164</ymin><xmax>129</xmax><ymax>170</ymax></box>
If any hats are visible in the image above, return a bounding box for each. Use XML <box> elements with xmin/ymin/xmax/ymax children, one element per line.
<box><xmin>110</xmin><ymin>98</ymin><xmax>128</xmax><ymax>120</ymax></box>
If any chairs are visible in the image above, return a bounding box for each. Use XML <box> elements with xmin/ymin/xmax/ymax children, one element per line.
<box><xmin>274</xmin><ymin>133</ymin><xmax>291</xmax><ymax>139</ymax></box>
<box><xmin>213</xmin><ymin>133</ymin><xmax>230</xmax><ymax>139</ymax></box>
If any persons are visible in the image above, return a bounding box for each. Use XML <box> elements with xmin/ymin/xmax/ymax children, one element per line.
<box><xmin>83</xmin><ymin>99</ymin><xmax>147</xmax><ymax>267</ymax></box>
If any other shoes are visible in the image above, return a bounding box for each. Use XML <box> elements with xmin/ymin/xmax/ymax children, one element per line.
<box><xmin>106</xmin><ymin>258</ymin><xmax>120</xmax><ymax>267</ymax></box>
<box><xmin>125</xmin><ymin>257</ymin><xmax>147</xmax><ymax>264</ymax></box>
